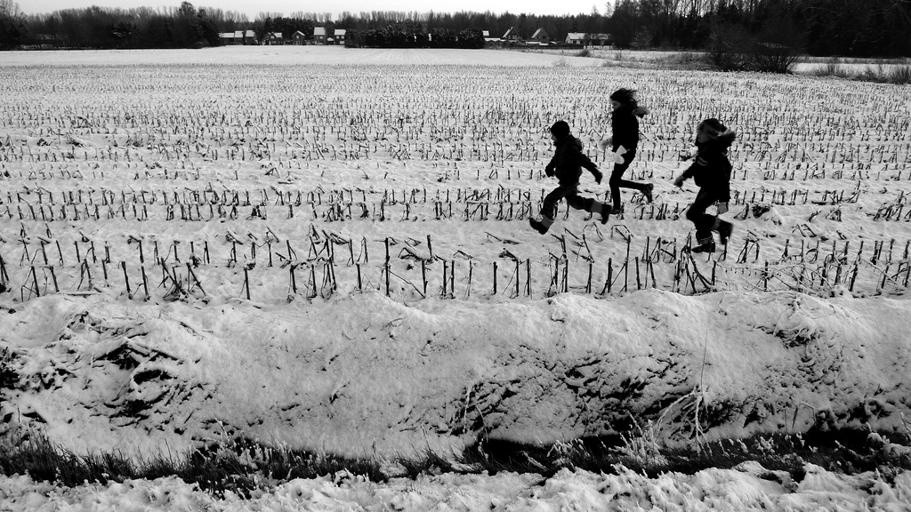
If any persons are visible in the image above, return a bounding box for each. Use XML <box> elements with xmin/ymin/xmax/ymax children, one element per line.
<box><xmin>601</xmin><ymin>87</ymin><xmax>654</xmax><ymax>215</ymax></box>
<box><xmin>529</xmin><ymin>121</ymin><xmax>612</xmax><ymax>234</ymax></box>
<box><xmin>673</xmin><ymin>118</ymin><xmax>738</xmax><ymax>254</ymax></box>
